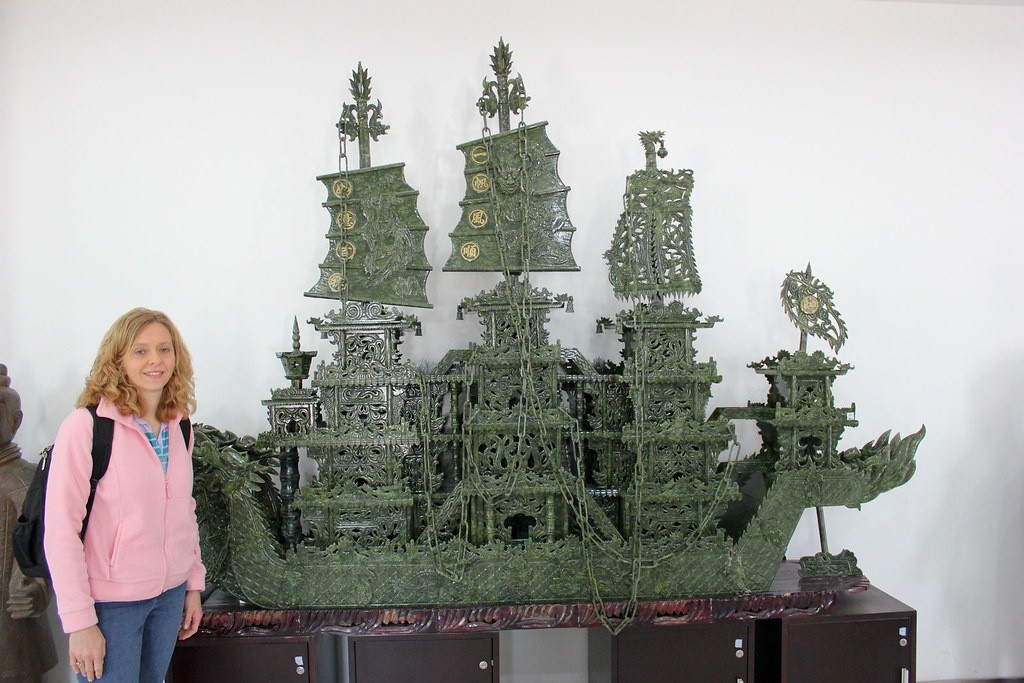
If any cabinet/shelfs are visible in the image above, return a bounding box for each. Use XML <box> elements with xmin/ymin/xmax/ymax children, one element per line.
<box><xmin>348</xmin><ymin>630</ymin><xmax>500</xmax><ymax>683</ymax></box>
<box><xmin>165</xmin><ymin>635</ymin><xmax>315</xmax><ymax>683</ymax></box>
<box><xmin>588</xmin><ymin>585</ymin><xmax>917</xmax><ymax>683</ymax></box>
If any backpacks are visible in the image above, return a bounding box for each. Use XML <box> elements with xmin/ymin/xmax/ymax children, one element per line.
<box><xmin>11</xmin><ymin>404</ymin><xmax>191</xmax><ymax>578</ymax></box>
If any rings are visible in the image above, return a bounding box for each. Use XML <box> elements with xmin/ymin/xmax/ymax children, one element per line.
<box><xmin>76</xmin><ymin>662</ymin><xmax>85</xmax><ymax>666</ymax></box>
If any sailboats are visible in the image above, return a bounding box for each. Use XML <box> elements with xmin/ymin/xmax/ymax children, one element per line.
<box><xmin>191</xmin><ymin>37</ymin><xmax>929</xmax><ymax>607</ymax></box>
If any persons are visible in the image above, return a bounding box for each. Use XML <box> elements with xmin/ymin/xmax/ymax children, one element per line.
<box><xmin>0</xmin><ymin>363</ymin><xmax>59</xmax><ymax>683</ymax></box>
<box><xmin>44</xmin><ymin>306</ymin><xmax>207</xmax><ymax>683</ymax></box>
